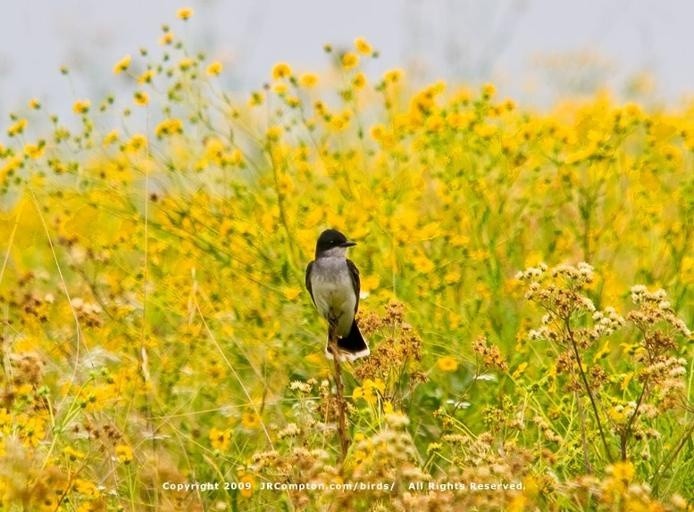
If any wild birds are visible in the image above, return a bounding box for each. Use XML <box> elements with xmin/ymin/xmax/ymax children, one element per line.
<box><xmin>305</xmin><ymin>229</ymin><xmax>370</xmax><ymax>362</ymax></box>
